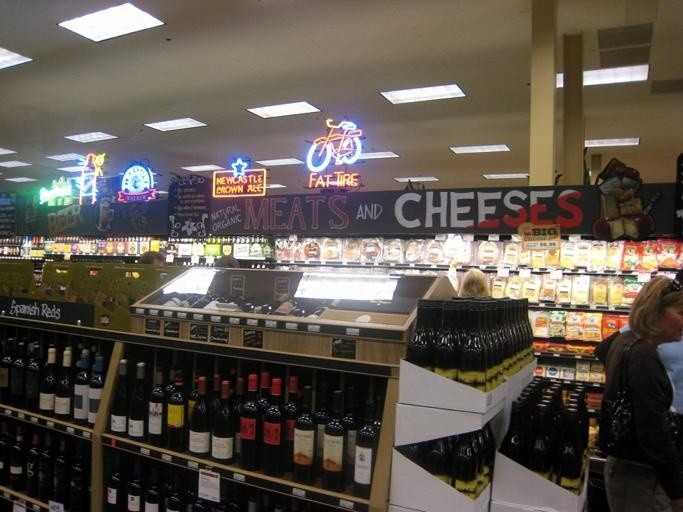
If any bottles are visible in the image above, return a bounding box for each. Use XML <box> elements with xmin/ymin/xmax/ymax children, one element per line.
<box><xmin>188</xmin><ymin>376</ymin><xmax>213</xmax><ymax>459</ymax></box>
<box><xmin>86</xmin><ymin>356</ymin><xmax>106</xmax><ymax>429</ymax></box>
<box><xmin>108</xmin><ymin>359</ymin><xmax>130</xmax><ymax>437</ymax></box>
<box><xmin>406</xmin><ymin>296</ymin><xmax>535</xmax><ymax>395</ymax></box>
<box><xmin>498</xmin><ymin>376</ymin><xmax>593</xmax><ymax>496</ymax></box>
<box><xmin>291</xmin><ymin>385</ymin><xmax>320</xmax><ymax>487</ymax></box>
<box><xmin>320</xmin><ymin>389</ymin><xmax>348</xmax><ymax>494</ymax></box>
<box><xmin>54</xmin><ymin>344</ymin><xmax>75</xmax><ymax>421</ymax></box>
<box><xmin>354</xmin><ymin>395</ymin><xmax>380</xmax><ymax>501</ymax></box>
<box><xmin>397</xmin><ymin>421</ymin><xmax>497</xmax><ymax>501</ymax></box>
<box><xmin>73</xmin><ymin>349</ymin><xmax>90</xmax><ymax>428</ymax></box>
<box><xmin>130</xmin><ymin>363</ymin><xmax>149</xmax><ymax>441</ymax></box>
<box><xmin>39</xmin><ymin>346</ymin><xmax>59</xmax><ymax>417</ymax></box>
<box><xmin>1</xmin><ymin>414</ymin><xmax>94</xmax><ymax>512</ymax></box>
<box><xmin>212</xmin><ymin>381</ymin><xmax>236</xmax><ymax>465</ymax></box>
<box><xmin>1</xmin><ymin>330</ymin><xmax>39</xmax><ymax>416</ymax></box>
<box><xmin>262</xmin><ymin>379</ymin><xmax>290</xmax><ymax>478</ymax></box>
<box><xmin>101</xmin><ymin>447</ymin><xmax>367</xmax><ymax>512</ymax></box>
<box><xmin>168</xmin><ymin>376</ymin><xmax>188</xmax><ymax>452</ymax></box>
<box><xmin>148</xmin><ymin>373</ymin><xmax>170</xmax><ymax>448</ymax></box>
<box><xmin>240</xmin><ymin>375</ymin><xmax>262</xmax><ymax>474</ymax></box>
<box><xmin>133</xmin><ymin>285</ymin><xmax>340</xmax><ymax>334</ymax></box>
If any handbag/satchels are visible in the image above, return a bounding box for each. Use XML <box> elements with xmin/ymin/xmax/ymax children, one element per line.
<box><xmin>599</xmin><ymin>339</ymin><xmax>683</xmax><ymax>462</ymax></box>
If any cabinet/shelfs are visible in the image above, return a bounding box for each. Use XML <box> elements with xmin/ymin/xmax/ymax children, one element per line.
<box><xmin>1</xmin><ymin>260</ymin><xmax>461</xmax><ymax>512</ymax></box>
<box><xmin>0</xmin><ymin>236</ymin><xmax>683</xmax><ymax>476</ymax></box>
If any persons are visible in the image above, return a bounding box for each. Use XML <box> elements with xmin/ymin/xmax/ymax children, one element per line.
<box><xmin>596</xmin><ymin>276</ymin><xmax>683</xmax><ymax>512</ymax></box>
<box><xmin>593</xmin><ymin>272</ymin><xmax>683</xmax><ymax>416</ymax></box>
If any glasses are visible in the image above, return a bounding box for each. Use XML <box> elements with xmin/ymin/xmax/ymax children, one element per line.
<box><xmin>660</xmin><ymin>279</ymin><xmax>681</xmax><ymax>296</ymax></box>
